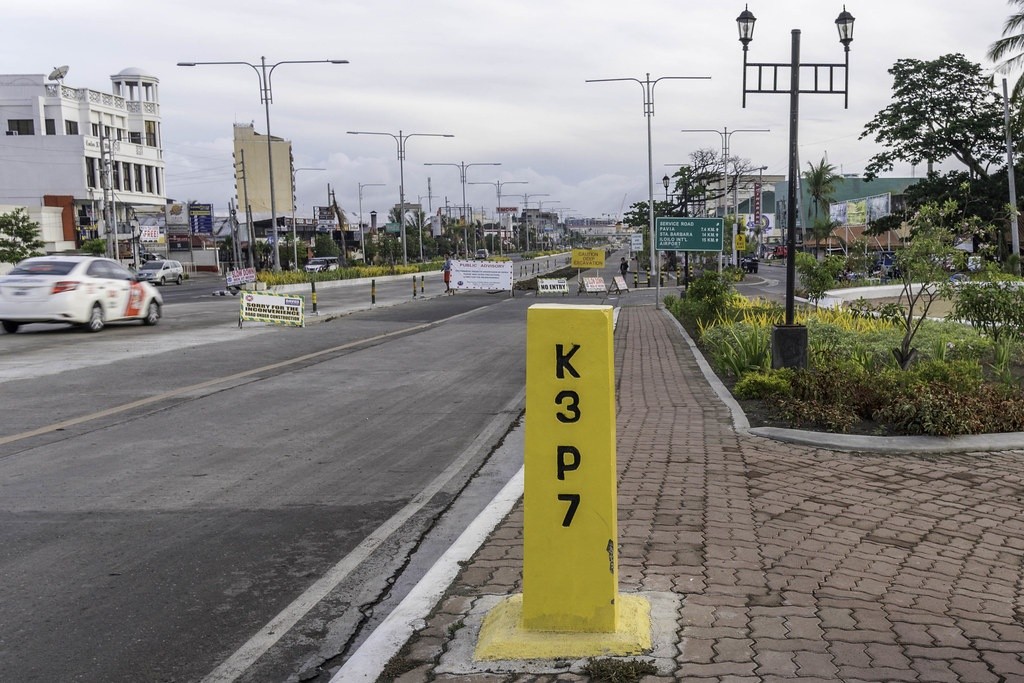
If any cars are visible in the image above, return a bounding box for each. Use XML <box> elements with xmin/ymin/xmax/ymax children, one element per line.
<box><xmin>543</xmin><ymin>244</ymin><xmax>584</xmax><ymax>250</ymax></box>
<box><xmin>305</xmin><ymin>257</ymin><xmax>339</xmax><ymax>273</ymax></box>
<box><xmin>135</xmin><ymin>259</ymin><xmax>184</xmax><ymax>285</ymax></box>
<box><xmin>0</xmin><ymin>256</ymin><xmax>163</xmax><ymax>333</ymax></box>
<box><xmin>738</xmin><ymin>252</ymin><xmax>759</xmax><ymax>273</ymax></box>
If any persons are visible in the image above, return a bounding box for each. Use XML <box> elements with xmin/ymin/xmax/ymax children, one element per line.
<box><xmin>441</xmin><ymin>252</ymin><xmax>452</xmax><ymax>292</ymax></box>
<box><xmin>620</xmin><ymin>257</ymin><xmax>628</xmax><ymax>282</ymax></box>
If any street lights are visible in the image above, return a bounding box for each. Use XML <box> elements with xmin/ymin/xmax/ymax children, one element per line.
<box><xmin>469</xmin><ymin>181</ymin><xmax>581</xmax><ymax>259</ymax></box>
<box><xmin>738</xmin><ymin>2</ymin><xmax>855</xmax><ymax>324</ymax></box>
<box><xmin>177</xmin><ymin>59</ymin><xmax>350</xmax><ymax>274</ymax></box>
<box><xmin>424</xmin><ymin>162</ymin><xmax>502</xmax><ymax>261</ymax></box>
<box><xmin>662</xmin><ymin>174</ymin><xmax>670</xmax><ymax>215</ymax></box>
<box><xmin>347</xmin><ymin>131</ymin><xmax>454</xmax><ymax>265</ymax></box>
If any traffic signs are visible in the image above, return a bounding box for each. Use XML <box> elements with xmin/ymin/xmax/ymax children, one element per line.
<box><xmin>655</xmin><ymin>217</ymin><xmax>722</xmax><ymax>251</ymax></box>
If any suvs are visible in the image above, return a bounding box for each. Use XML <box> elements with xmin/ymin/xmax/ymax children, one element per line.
<box><xmin>762</xmin><ymin>245</ymin><xmax>788</xmax><ymax>260</ymax></box>
<box><xmin>475</xmin><ymin>249</ymin><xmax>488</xmax><ymax>259</ymax></box>
<box><xmin>873</xmin><ymin>252</ymin><xmax>896</xmax><ymax>271</ymax></box>
